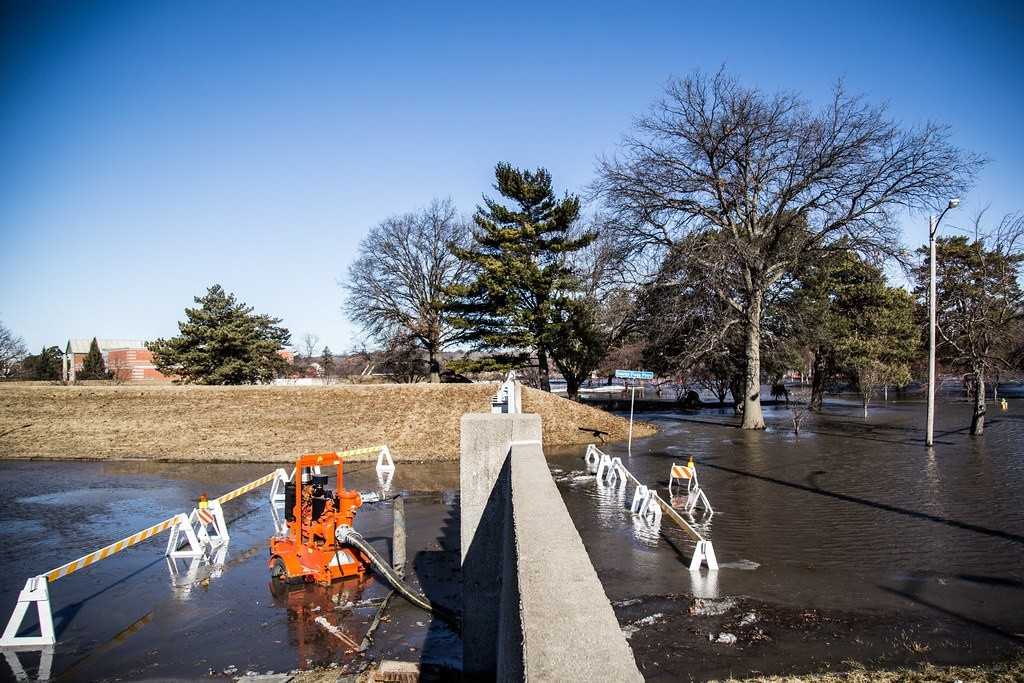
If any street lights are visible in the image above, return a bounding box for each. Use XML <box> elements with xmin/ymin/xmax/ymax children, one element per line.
<box><xmin>924</xmin><ymin>198</ymin><xmax>960</xmax><ymax>447</ymax></box>
